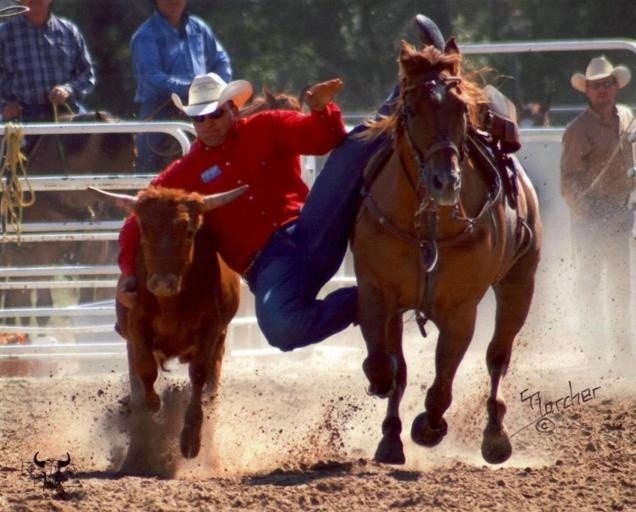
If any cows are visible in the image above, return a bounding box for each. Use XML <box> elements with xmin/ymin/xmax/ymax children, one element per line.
<box><xmin>87</xmin><ymin>183</ymin><xmax>251</xmax><ymax>475</ymax></box>
<box><xmin>33</xmin><ymin>452</ymin><xmax>69</xmax><ymax>489</ymax></box>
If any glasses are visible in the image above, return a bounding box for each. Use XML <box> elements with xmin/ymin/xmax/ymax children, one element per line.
<box><xmin>191</xmin><ymin>108</ymin><xmax>224</xmax><ymax>123</ymax></box>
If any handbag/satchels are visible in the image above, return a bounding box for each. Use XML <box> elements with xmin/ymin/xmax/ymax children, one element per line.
<box><xmin>415</xmin><ymin>13</ymin><xmax>445</xmax><ymax>51</ymax></box>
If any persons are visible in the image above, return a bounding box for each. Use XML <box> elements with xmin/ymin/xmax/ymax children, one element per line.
<box><xmin>114</xmin><ymin>12</ymin><xmax>447</xmax><ymax>355</ymax></box>
<box><xmin>0</xmin><ymin>0</ymin><xmax>97</xmax><ymax>157</ymax></box>
<box><xmin>128</xmin><ymin>0</ymin><xmax>233</xmax><ymax>173</ymax></box>
<box><xmin>558</xmin><ymin>54</ymin><xmax>636</xmax><ymax>361</ymax></box>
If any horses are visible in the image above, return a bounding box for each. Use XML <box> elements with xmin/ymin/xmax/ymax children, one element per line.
<box><xmin>97</xmin><ymin>85</ymin><xmax>314</xmax><ymax>349</ymax></box>
<box><xmin>352</xmin><ymin>36</ymin><xmax>543</xmax><ymax>465</ymax></box>
<box><xmin>512</xmin><ymin>88</ymin><xmax>554</xmax><ymax>129</ymax></box>
<box><xmin>0</xmin><ymin>97</ymin><xmax>143</xmax><ymax>345</ymax></box>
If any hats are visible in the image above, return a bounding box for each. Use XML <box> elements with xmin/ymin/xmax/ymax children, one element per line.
<box><xmin>171</xmin><ymin>71</ymin><xmax>253</xmax><ymax>117</ymax></box>
<box><xmin>569</xmin><ymin>55</ymin><xmax>631</xmax><ymax>93</ymax></box>
